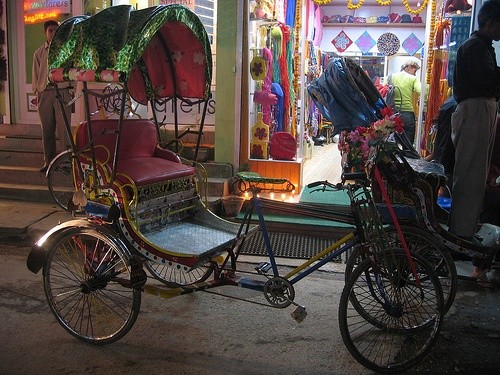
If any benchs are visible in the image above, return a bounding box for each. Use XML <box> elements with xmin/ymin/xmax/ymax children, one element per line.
<box><xmin>376</xmin><ymin>126</ymin><xmax>448</xmax><ymax>191</ymax></box>
<box><xmin>71</xmin><ymin>118</ymin><xmax>197</xmax><ymax>189</ymax></box>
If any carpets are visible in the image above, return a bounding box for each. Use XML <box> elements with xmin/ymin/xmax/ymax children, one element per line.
<box><xmin>299</xmin><ymin>185</ymin><xmax>373</xmax><ymax>208</ymax></box>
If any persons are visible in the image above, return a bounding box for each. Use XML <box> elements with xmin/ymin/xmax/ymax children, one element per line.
<box><xmin>31</xmin><ymin>20</ymin><xmax>75</xmax><ymax>172</ymax></box>
<box><xmin>385</xmin><ymin>60</ymin><xmax>421</xmax><ymax>150</ymax></box>
<box><xmin>432</xmin><ymin>96</ymin><xmax>500</xmax><ymax>193</ymax></box>
<box><xmin>447</xmin><ymin>0</ymin><xmax>500</xmax><ymax>243</ymax></box>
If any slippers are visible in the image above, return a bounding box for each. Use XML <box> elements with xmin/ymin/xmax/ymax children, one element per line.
<box><xmin>489</xmin><ymin>274</ymin><xmax>500</xmax><ymax>288</ymax></box>
<box><xmin>472</xmin><ymin>274</ymin><xmax>495</xmax><ymax>288</ymax></box>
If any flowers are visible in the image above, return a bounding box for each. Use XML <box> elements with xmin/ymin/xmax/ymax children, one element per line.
<box><xmin>333</xmin><ymin>76</ymin><xmax>403</xmax><ymax>168</ymax></box>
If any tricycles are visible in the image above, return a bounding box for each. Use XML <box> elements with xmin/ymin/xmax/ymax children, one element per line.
<box><xmin>31</xmin><ymin>2</ymin><xmax>463</xmax><ymax>373</ymax></box>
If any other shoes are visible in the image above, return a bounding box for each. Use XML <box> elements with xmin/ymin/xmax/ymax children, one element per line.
<box><xmin>458</xmin><ymin>234</ymin><xmax>484</xmax><ymax>245</ymax></box>
<box><xmin>39</xmin><ymin>165</ymin><xmax>58</xmax><ymax>172</ymax></box>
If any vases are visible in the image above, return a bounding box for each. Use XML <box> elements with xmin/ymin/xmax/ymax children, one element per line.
<box><xmin>221</xmin><ymin>196</ymin><xmax>246</xmax><ymax>217</ymax></box>
